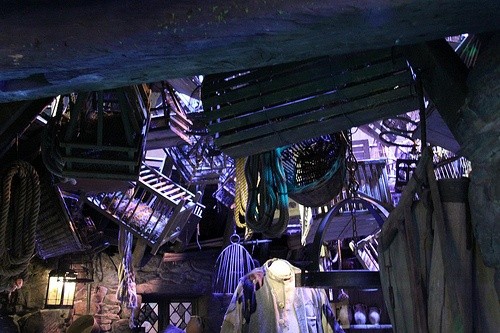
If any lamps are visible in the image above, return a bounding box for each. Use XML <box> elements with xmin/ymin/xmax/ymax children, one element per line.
<box><xmin>40</xmin><ymin>254</ymin><xmax>79</xmax><ymax>309</ymax></box>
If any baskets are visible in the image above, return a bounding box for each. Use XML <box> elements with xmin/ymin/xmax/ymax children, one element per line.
<box><xmin>278</xmin><ymin>131</ymin><xmax>348</xmax><ymax>209</ymax></box>
